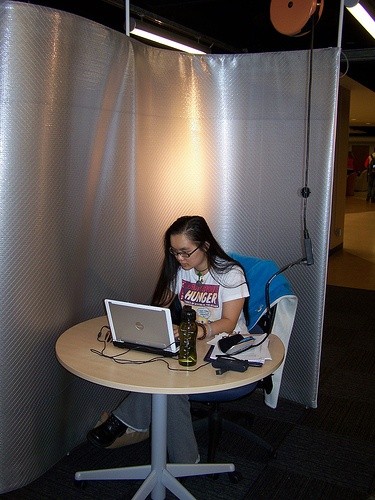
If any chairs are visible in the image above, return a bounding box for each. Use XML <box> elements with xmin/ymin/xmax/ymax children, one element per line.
<box><xmin>181</xmin><ymin>248</ymin><xmax>297</xmax><ymax>482</ymax></box>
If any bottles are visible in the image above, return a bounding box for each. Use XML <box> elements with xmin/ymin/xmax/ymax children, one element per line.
<box><xmin>178</xmin><ymin>307</ymin><xmax>198</xmax><ymax>366</ymax></box>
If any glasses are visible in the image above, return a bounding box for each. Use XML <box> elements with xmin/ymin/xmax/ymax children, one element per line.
<box><xmin>168</xmin><ymin>243</ymin><xmax>204</xmax><ymax>258</ymax></box>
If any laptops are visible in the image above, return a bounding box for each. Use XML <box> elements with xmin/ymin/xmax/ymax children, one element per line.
<box><xmin>104</xmin><ymin>298</ymin><xmax>182</xmax><ymax>357</ymax></box>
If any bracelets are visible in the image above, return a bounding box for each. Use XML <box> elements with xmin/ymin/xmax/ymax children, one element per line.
<box><xmin>198</xmin><ymin>323</ymin><xmax>207</xmax><ymax>340</ymax></box>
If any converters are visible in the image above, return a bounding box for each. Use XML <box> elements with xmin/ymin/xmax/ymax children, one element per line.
<box><xmin>212</xmin><ymin>358</ymin><xmax>248</xmax><ymax>372</ymax></box>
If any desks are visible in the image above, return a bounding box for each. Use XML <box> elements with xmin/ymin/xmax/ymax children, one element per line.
<box><xmin>55</xmin><ymin>315</ymin><xmax>286</xmax><ymax>500</ymax></box>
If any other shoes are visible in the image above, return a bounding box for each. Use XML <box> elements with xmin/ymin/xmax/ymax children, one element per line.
<box><xmin>87</xmin><ymin>414</ymin><xmax>128</xmax><ymax>448</ymax></box>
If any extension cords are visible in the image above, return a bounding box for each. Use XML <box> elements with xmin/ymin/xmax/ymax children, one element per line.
<box><xmin>304</xmin><ymin>238</ymin><xmax>314</xmax><ymax>265</ymax></box>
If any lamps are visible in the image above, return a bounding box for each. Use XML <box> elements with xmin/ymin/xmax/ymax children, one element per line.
<box><xmin>123</xmin><ymin>0</ymin><xmax>213</xmax><ymax>57</ymax></box>
<box><xmin>344</xmin><ymin>0</ymin><xmax>375</xmax><ymax>41</ymax></box>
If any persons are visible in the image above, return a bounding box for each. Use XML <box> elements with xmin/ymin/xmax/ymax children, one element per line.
<box><xmin>88</xmin><ymin>215</ymin><xmax>250</xmax><ymax>464</ymax></box>
